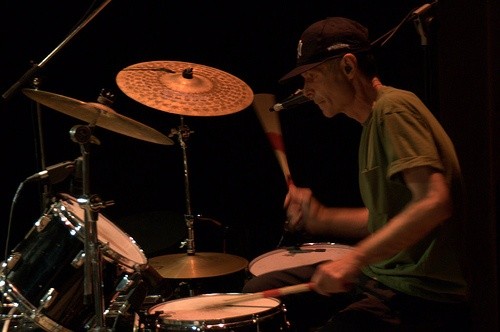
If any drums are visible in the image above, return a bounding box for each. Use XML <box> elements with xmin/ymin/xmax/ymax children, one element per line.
<box><xmin>1</xmin><ymin>192</ymin><xmax>150</xmax><ymax>332</ymax></box>
<box><xmin>143</xmin><ymin>292</ymin><xmax>290</xmax><ymax>332</ymax></box>
<box><xmin>244</xmin><ymin>242</ymin><xmax>361</xmax><ymax>280</ymax></box>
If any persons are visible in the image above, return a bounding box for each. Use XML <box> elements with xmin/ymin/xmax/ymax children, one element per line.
<box><xmin>282</xmin><ymin>17</ymin><xmax>474</xmax><ymax>332</ymax></box>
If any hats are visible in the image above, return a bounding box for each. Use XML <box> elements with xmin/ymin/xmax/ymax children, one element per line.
<box><xmin>279</xmin><ymin>16</ymin><xmax>371</xmax><ymax>83</ymax></box>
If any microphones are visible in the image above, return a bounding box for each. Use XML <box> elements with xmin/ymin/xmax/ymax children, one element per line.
<box><xmin>24</xmin><ymin>161</ymin><xmax>80</xmax><ymax>186</ymax></box>
<box><xmin>414</xmin><ymin>15</ymin><xmax>433</xmax><ymax>46</ymax></box>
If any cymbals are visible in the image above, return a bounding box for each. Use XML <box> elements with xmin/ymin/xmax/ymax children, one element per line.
<box><xmin>21</xmin><ymin>86</ymin><xmax>178</xmax><ymax>146</ymax></box>
<box><xmin>114</xmin><ymin>59</ymin><xmax>255</xmax><ymax>118</ymax></box>
<box><xmin>146</xmin><ymin>250</ymin><xmax>250</xmax><ymax>280</ymax></box>
